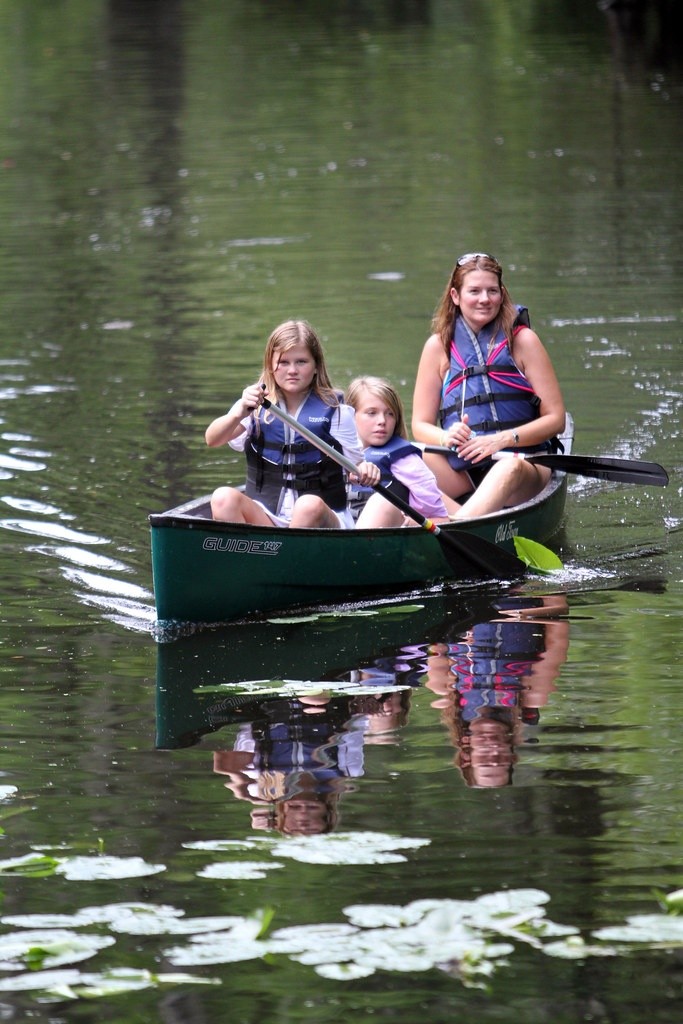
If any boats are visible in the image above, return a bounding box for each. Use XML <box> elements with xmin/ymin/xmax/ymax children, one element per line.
<box><xmin>156</xmin><ymin>533</ymin><xmax>563</xmax><ymax>749</ymax></box>
<box><xmin>147</xmin><ymin>409</ymin><xmax>574</xmax><ymax>622</ymax></box>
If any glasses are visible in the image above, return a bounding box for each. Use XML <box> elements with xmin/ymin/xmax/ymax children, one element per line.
<box><xmin>457</xmin><ymin>252</ymin><xmax>498</xmax><ymax>266</ymax></box>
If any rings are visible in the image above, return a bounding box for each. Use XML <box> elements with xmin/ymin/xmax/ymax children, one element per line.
<box><xmin>476</xmin><ymin>448</ymin><xmax>484</xmax><ymax>453</ymax></box>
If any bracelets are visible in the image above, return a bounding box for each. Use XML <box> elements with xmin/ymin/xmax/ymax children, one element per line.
<box><xmin>509</xmin><ymin>428</ymin><xmax>520</xmax><ymax>447</ymax></box>
<box><xmin>438</xmin><ymin>430</ymin><xmax>448</xmax><ymax>447</ymax></box>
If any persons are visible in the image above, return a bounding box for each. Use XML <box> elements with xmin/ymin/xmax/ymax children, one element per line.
<box><xmin>206</xmin><ymin>321</ymin><xmax>382</xmax><ymax>529</ymax></box>
<box><xmin>410</xmin><ymin>252</ymin><xmax>566</xmax><ymax>518</ymax></box>
<box><xmin>345</xmin><ymin>374</ymin><xmax>451</xmax><ymax>527</ymax></box>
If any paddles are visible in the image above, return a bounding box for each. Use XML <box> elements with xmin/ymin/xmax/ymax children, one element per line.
<box><xmin>261</xmin><ymin>398</ymin><xmax>564</xmax><ymax>580</ymax></box>
<box><xmin>409</xmin><ymin>440</ymin><xmax>668</xmax><ymax>490</ymax></box>
